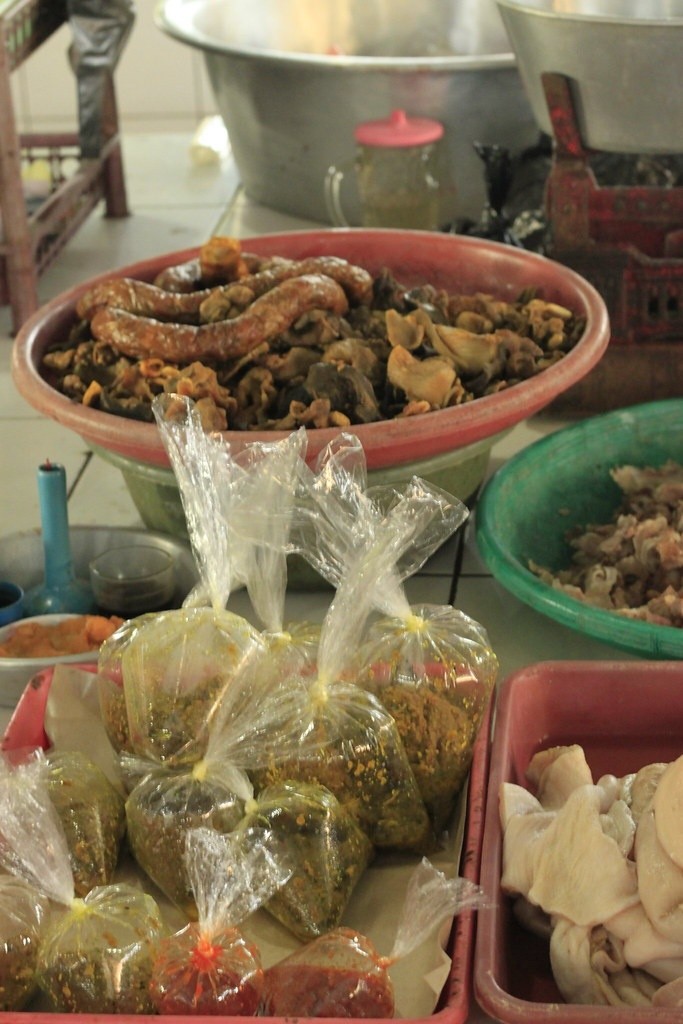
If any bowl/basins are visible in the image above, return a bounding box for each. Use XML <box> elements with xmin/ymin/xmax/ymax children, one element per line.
<box><xmin>499</xmin><ymin>2</ymin><xmax>683</xmax><ymax>151</ymax></box>
<box><xmin>151</xmin><ymin>1</ymin><xmax>543</xmax><ymax>229</ymax></box>
<box><xmin>11</xmin><ymin>228</ymin><xmax>683</xmax><ymax>660</ymax></box>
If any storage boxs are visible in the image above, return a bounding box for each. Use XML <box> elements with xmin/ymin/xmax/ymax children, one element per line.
<box><xmin>0</xmin><ymin>658</ymin><xmax>496</xmax><ymax>1024</ymax></box>
<box><xmin>475</xmin><ymin>658</ymin><xmax>683</xmax><ymax>1023</ymax></box>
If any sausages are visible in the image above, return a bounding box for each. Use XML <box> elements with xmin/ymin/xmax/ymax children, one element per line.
<box><xmin>72</xmin><ymin>231</ymin><xmax>373</xmax><ymax>359</ymax></box>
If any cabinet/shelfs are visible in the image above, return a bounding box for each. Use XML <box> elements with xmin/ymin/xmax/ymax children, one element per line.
<box><xmin>0</xmin><ymin>0</ymin><xmax>136</xmax><ymax>338</ymax></box>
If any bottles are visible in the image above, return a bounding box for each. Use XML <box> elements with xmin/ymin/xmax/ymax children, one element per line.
<box><xmin>24</xmin><ymin>462</ymin><xmax>92</xmax><ymax>618</ymax></box>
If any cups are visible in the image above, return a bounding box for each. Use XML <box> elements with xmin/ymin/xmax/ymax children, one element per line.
<box><xmin>324</xmin><ymin>110</ymin><xmax>442</xmax><ymax>235</ymax></box>
<box><xmin>92</xmin><ymin>546</ymin><xmax>176</xmax><ymax>616</ymax></box>
<box><xmin>0</xmin><ymin>581</ymin><xmax>23</xmax><ymax>628</ymax></box>
